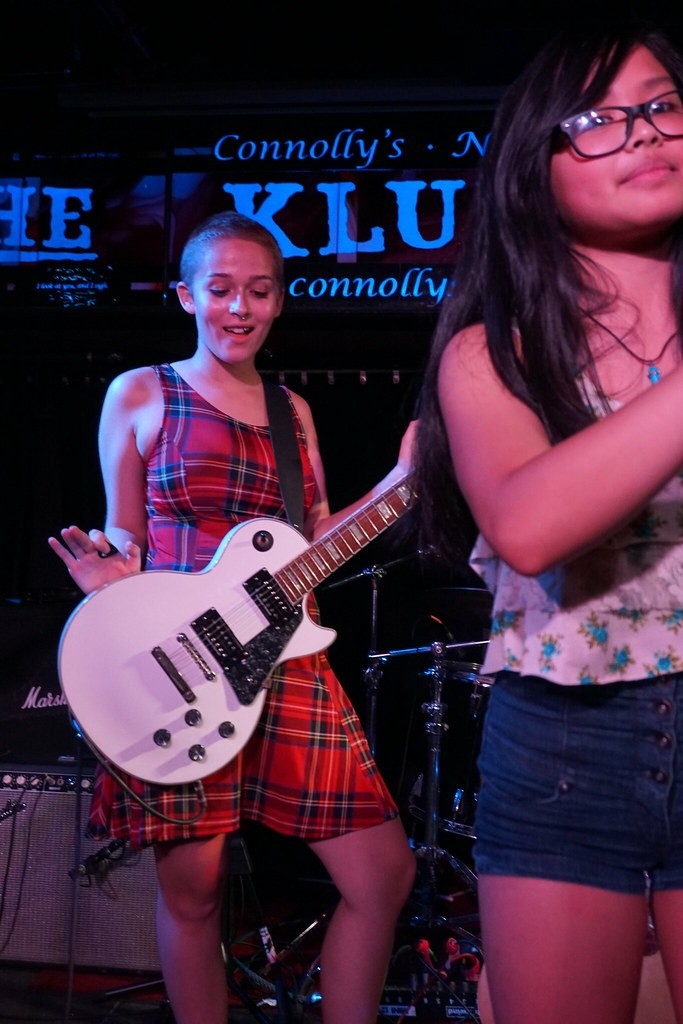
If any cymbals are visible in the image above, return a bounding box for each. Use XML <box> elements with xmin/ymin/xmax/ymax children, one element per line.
<box><xmin>432</xmin><ymin>586</ymin><xmax>493</xmax><ymax>603</ymax></box>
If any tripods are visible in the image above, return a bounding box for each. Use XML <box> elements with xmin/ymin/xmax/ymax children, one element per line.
<box><xmin>269</xmin><ymin>640</ymin><xmax>492</xmax><ymax>1000</ymax></box>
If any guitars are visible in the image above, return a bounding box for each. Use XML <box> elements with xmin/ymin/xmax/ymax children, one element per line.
<box><xmin>56</xmin><ymin>471</ymin><xmax>421</xmax><ymax>787</ymax></box>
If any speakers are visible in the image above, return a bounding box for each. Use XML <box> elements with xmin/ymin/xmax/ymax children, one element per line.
<box><xmin>0</xmin><ymin>763</ymin><xmax>164</xmax><ymax>980</ymax></box>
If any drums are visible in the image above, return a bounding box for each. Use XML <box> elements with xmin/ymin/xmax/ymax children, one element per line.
<box><xmin>394</xmin><ymin>658</ymin><xmax>497</xmax><ymax>842</ymax></box>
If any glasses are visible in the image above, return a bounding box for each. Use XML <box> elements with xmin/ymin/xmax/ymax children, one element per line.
<box><xmin>558</xmin><ymin>90</ymin><xmax>683</xmax><ymax>158</ymax></box>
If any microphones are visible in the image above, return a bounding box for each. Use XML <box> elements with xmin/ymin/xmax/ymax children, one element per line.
<box><xmin>77</xmin><ymin>855</ymin><xmax>102</xmax><ymax>876</ymax></box>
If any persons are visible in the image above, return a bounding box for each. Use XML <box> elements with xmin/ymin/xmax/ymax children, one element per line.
<box><xmin>48</xmin><ymin>211</ymin><xmax>421</xmax><ymax>1024</ymax></box>
<box><xmin>420</xmin><ymin>19</ymin><xmax>683</xmax><ymax>1024</ymax></box>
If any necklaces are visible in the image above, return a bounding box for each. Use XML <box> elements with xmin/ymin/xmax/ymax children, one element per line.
<box><xmin>575</xmin><ymin>302</ymin><xmax>682</xmax><ymax>385</ymax></box>
